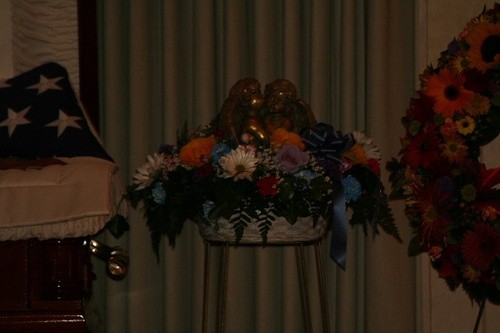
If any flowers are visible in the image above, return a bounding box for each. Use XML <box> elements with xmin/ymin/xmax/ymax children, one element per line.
<box><xmin>122</xmin><ymin>119</ymin><xmax>407</xmax><ymax>243</ymax></box>
<box><xmin>381</xmin><ymin>1</ymin><xmax>499</xmax><ymax>305</ymax></box>
